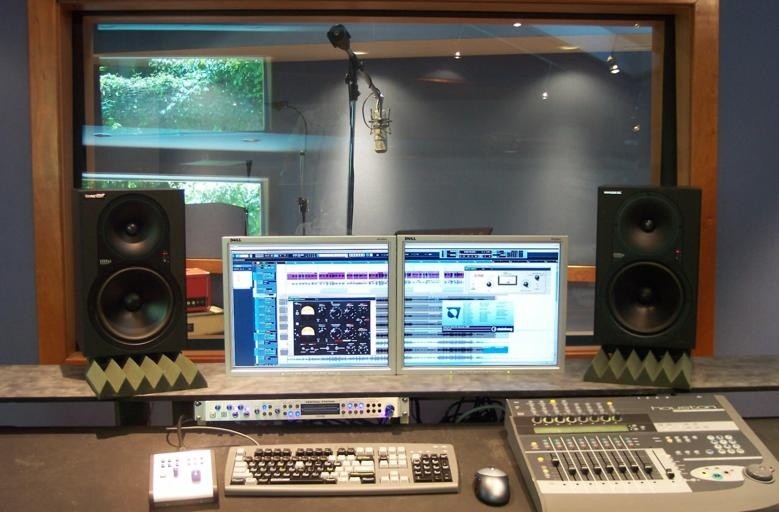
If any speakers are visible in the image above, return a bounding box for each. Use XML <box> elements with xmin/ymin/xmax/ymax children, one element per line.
<box><xmin>73</xmin><ymin>189</ymin><xmax>187</xmax><ymax>360</ymax></box>
<box><xmin>593</xmin><ymin>186</ymin><xmax>701</xmax><ymax>352</ymax></box>
<box><xmin>185</xmin><ymin>267</ymin><xmax>211</xmax><ymax>311</ymax></box>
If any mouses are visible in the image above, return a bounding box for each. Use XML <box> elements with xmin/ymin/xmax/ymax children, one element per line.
<box><xmin>476</xmin><ymin>468</ymin><xmax>510</xmax><ymax>506</ymax></box>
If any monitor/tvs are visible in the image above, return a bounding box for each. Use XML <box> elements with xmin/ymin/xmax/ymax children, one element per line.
<box><xmin>221</xmin><ymin>234</ymin><xmax>395</xmax><ymax>374</ymax></box>
<box><xmin>396</xmin><ymin>235</ymin><xmax>566</xmax><ymax>377</ymax></box>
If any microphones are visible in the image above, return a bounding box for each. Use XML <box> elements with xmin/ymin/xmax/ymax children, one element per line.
<box><xmin>371</xmin><ymin>100</ymin><xmax>392</xmax><ymax>152</ymax></box>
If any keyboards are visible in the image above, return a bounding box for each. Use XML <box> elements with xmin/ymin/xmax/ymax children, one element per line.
<box><xmin>225</xmin><ymin>443</ymin><xmax>459</xmax><ymax>495</ymax></box>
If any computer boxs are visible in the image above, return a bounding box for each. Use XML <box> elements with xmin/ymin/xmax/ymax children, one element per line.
<box><xmin>193</xmin><ymin>397</ymin><xmax>410</xmax><ymax>424</ymax></box>
